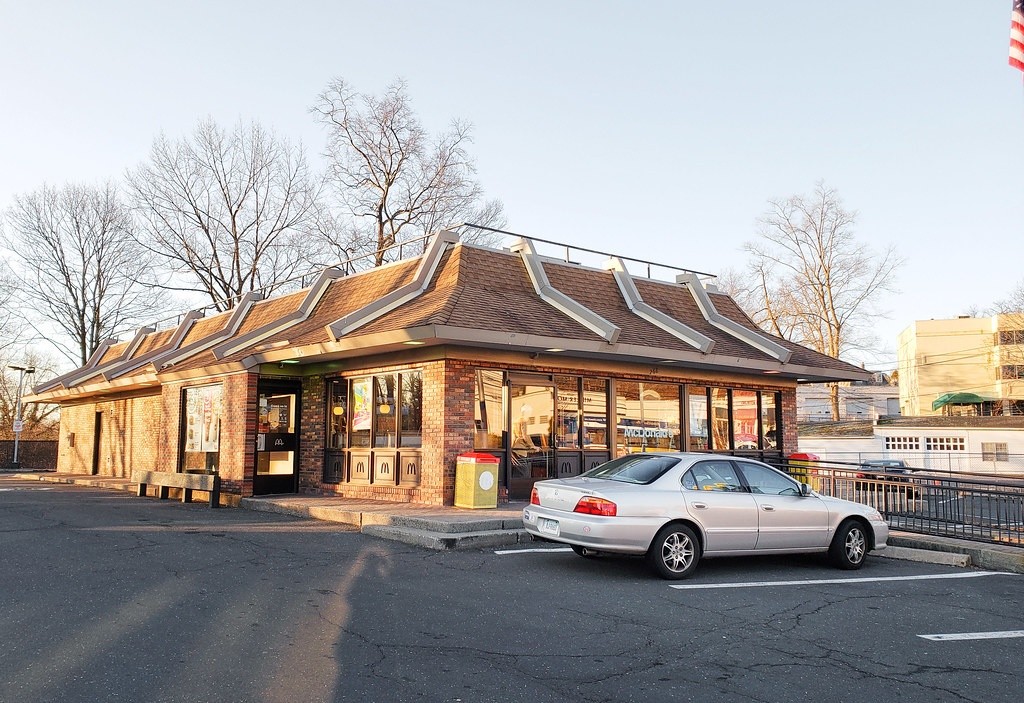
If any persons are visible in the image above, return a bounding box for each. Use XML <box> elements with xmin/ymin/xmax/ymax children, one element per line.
<box><xmin>511</xmin><ymin>406</ymin><xmax>540</xmax><ymax>478</ymax></box>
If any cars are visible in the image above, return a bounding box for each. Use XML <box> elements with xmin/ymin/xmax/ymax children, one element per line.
<box><xmin>522</xmin><ymin>453</ymin><xmax>890</xmax><ymax>581</ymax></box>
<box><xmin>855</xmin><ymin>459</ymin><xmax>917</xmax><ymax>500</ymax></box>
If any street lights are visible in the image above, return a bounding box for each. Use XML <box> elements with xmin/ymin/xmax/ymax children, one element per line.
<box><xmin>8</xmin><ymin>365</ymin><xmax>35</xmax><ymax>469</ymax></box>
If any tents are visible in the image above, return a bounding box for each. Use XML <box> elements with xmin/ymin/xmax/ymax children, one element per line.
<box><xmin>931</xmin><ymin>391</ymin><xmax>985</xmax><ymax>416</ymax></box>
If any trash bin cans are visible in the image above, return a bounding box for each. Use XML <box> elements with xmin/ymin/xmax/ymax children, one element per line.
<box><xmin>787</xmin><ymin>452</ymin><xmax>820</xmax><ymax>495</ymax></box>
<box><xmin>453</xmin><ymin>452</ymin><xmax>501</xmax><ymax>509</ymax></box>
